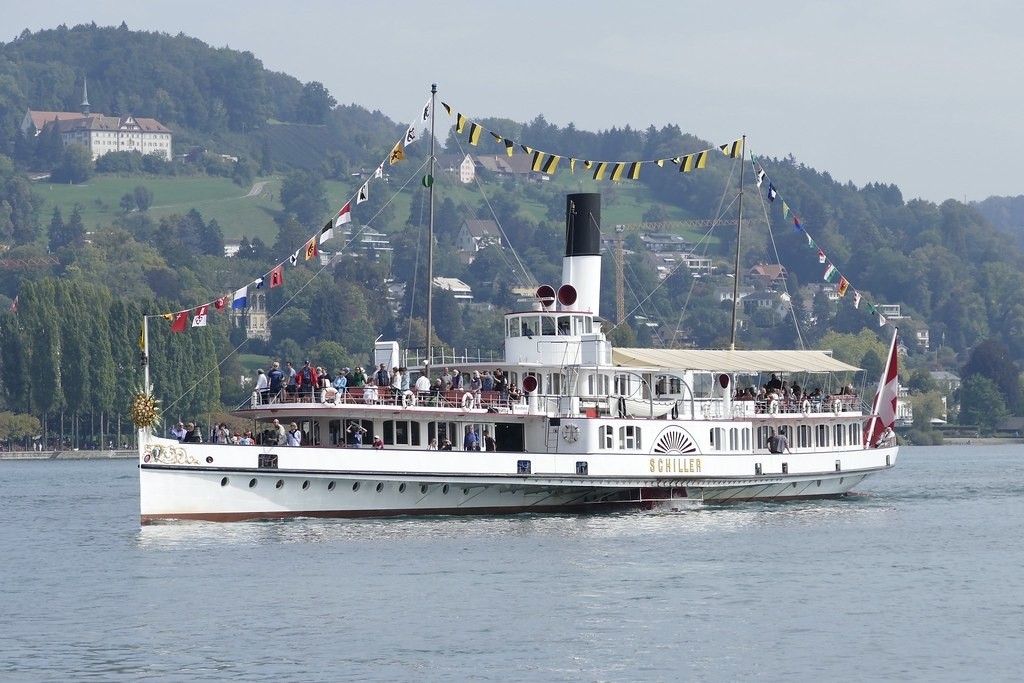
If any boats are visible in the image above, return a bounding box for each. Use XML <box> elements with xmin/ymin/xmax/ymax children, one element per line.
<box><xmin>606</xmin><ymin>395</ymin><xmax>677</xmax><ymax>419</ymax></box>
<box><xmin>135</xmin><ymin>83</ymin><xmax>900</xmax><ymax>524</ymax></box>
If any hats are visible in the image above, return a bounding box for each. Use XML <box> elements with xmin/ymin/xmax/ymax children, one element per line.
<box><xmin>305</xmin><ymin>360</ymin><xmax>309</xmax><ymax>364</ymax></box>
<box><xmin>343</xmin><ymin>367</ymin><xmax>350</xmax><ymax>371</ymax></box>
<box><xmin>374</xmin><ymin>435</ymin><xmax>379</xmax><ymax>438</ymax></box>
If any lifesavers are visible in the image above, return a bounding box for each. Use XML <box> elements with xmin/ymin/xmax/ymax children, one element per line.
<box><xmin>770</xmin><ymin>400</ymin><xmax>778</xmax><ymax>417</ymax></box>
<box><xmin>561</xmin><ymin>423</ymin><xmax>580</xmax><ymax>443</ymax></box>
<box><xmin>803</xmin><ymin>400</ymin><xmax>810</xmax><ymax>417</ymax></box>
<box><xmin>321</xmin><ymin>387</ymin><xmax>340</xmax><ymax>407</ymax></box>
<box><xmin>402</xmin><ymin>390</ymin><xmax>415</xmax><ymax>410</ymax></box>
<box><xmin>834</xmin><ymin>398</ymin><xmax>842</xmax><ymax>416</ymax></box>
<box><xmin>461</xmin><ymin>392</ymin><xmax>473</xmax><ymax>412</ymax></box>
<box><xmin>251</xmin><ymin>391</ymin><xmax>257</xmax><ymax>407</ymax></box>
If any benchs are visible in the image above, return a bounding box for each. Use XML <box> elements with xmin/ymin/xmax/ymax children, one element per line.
<box><xmin>824</xmin><ymin>395</ymin><xmax>859</xmax><ymax>409</ymax></box>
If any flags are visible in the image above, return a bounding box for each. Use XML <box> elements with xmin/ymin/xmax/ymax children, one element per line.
<box><xmin>137</xmin><ymin>319</ymin><xmax>145</xmax><ymax>353</ymax></box>
<box><xmin>862</xmin><ymin>337</ymin><xmax>900</xmax><ymax>447</ymax></box>
<box><xmin>162</xmin><ymin>98</ymin><xmax>888</xmax><ymax>334</ymax></box>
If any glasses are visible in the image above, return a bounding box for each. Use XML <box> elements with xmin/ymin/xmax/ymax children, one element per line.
<box><xmin>444</xmin><ymin>441</ymin><xmax>448</xmax><ymax>443</ymax></box>
<box><xmin>473</xmin><ymin>373</ymin><xmax>476</xmax><ymax>374</ymax></box>
<box><xmin>375</xmin><ymin>438</ymin><xmax>379</xmax><ymax>439</ymax></box>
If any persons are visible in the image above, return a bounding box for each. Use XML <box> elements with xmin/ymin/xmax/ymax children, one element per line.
<box><xmin>874</xmin><ymin>426</ymin><xmax>896</xmax><ymax>449</ymax></box>
<box><xmin>732</xmin><ymin>373</ymin><xmax>857</xmax><ymax>453</ymax></box>
<box><xmin>168</xmin><ymin>360</ymin><xmax>522</xmax><ymax>451</ymax></box>
<box><xmin>32</xmin><ymin>436</ymin><xmax>133</xmax><ymax>452</ymax></box>
